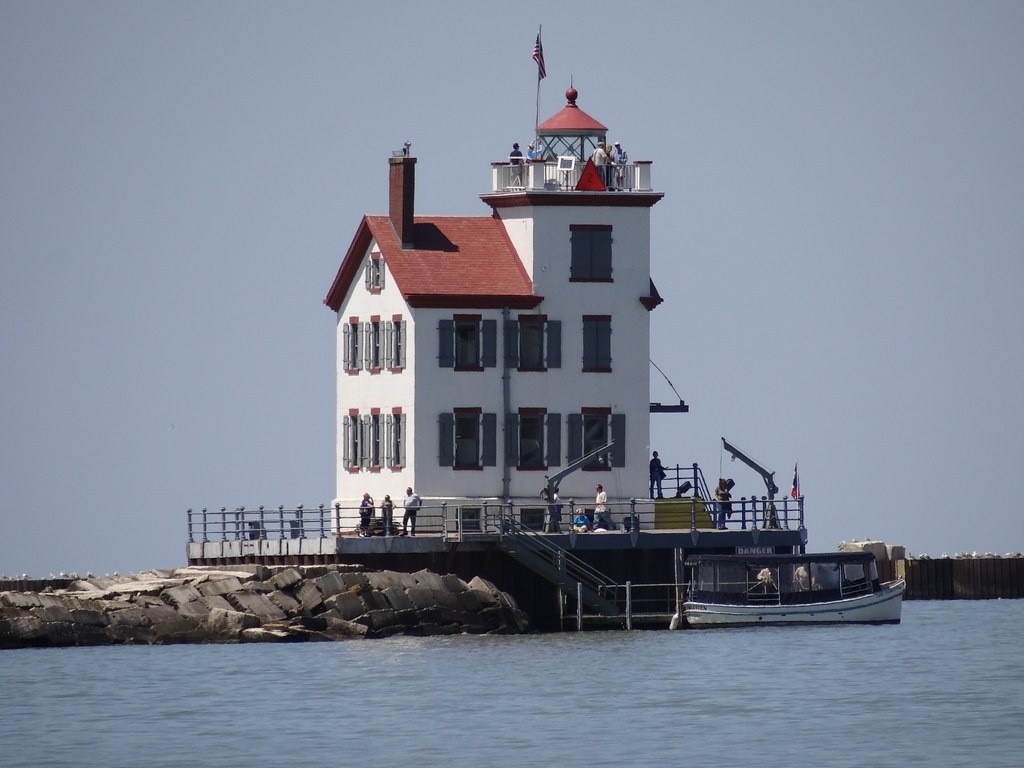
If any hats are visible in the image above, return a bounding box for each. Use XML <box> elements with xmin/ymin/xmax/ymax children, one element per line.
<box><xmin>598</xmin><ymin>141</ymin><xmax>605</xmax><ymax>146</ymax></box>
<box><xmin>614</xmin><ymin>142</ymin><xmax>621</xmax><ymax>145</ymax></box>
<box><xmin>513</xmin><ymin>143</ymin><xmax>520</xmax><ymax>149</ymax></box>
<box><xmin>527</xmin><ymin>144</ymin><xmax>534</xmax><ymax>151</ymax></box>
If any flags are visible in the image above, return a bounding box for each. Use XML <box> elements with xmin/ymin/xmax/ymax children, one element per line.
<box><xmin>532</xmin><ymin>31</ymin><xmax>547</xmax><ymax>80</ymax></box>
<box><xmin>791</xmin><ymin>462</ymin><xmax>800</xmax><ymax>501</ymax></box>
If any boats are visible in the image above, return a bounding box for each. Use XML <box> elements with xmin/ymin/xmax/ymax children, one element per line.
<box><xmin>683</xmin><ymin>552</ymin><xmax>907</xmax><ymax>630</ymax></box>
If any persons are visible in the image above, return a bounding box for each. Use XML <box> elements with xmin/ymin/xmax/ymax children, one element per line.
<box><xmin>795</xmin><ymin>563</ymin><xmax>816</xmax><ymax>591</ymax></box>
<box><xmin>358</xmin><ymin>493</ymin><xmax>374</xmax><ymax>537</ymax></box>
<box><xmin>593</xmin><ymin>142</ymin><xmax>619</xmax><ymax>185</ymax></box>
<box><xmin>509</xmin><ymin>143</ymin><xmax>524</xmax><ymax>192</ymax></box>
<box><xmin>573</xmin><ymin>508</ymin><xmax>589</xmax><ymax>533</ymax></box>
<box><xmin>756</xmin><ymin>567</ymin><xmax>773</xmax><ymax>593</ymax></box>
<box><xmin>610</xmin><ymin>142</ymin><xmax>628</xmax><ymax>192</ymax></box>
<box><xmin>649</xmin><ymin>451</ymin><xmax>668</xmax><ymax>498</ymax></box>
<box><xmin>554</xmin><ymin>487</ymin><xmax>562</xmax><ymax>532</ymax></box>
<box><xmin>591</xmin><ymin>484</ymin><xmax>616</xmax><ymax>532</ymax></box>
<box><xmin>398</xmin><ymin>487</ymin><xmax>422</xmax><ymax>537</ymax></box>
<box><xmin>381</xmin><ymin>495</ymin><xmax>396</xmax><ymax>536</ymax></box>
<box><xmin>605</xmin><ymin>144</ymin><xmax>613</xmax><ymax>187</ymax></box>
<box><xmin>714</xmin><ymin>478</ymin><xmax>728</xmax><ymax>530</ymax></box>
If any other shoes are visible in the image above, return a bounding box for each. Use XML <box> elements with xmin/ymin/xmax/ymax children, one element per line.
<box><xmin>398</xmin><ymin>531</ymin><xmax>408</xmax><ymax>536</ymax></box>
<box><xmin>718</xmin><ymin>527</ymin><xmax>728</xmax><ymax>530</ymax></box>
<box><xmin>411</xmin><ymin>533</ymin><xmax>415</xmax><ymax>536</ymax></box>
<box><xmin>613</xmin><ymin>523</ymin><xmax>616</xmax><ymax>530</ymax></box>
<box><xmin>358</xmin><ymin>532</ymin><xmax>371</xmax><ymax>537</ymax></box>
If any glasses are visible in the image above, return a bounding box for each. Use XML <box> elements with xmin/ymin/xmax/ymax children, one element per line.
<box><xmin>518</xmin><ymin>146</ymin><xmax>519</xmax><ymax>147</ymax></box>
